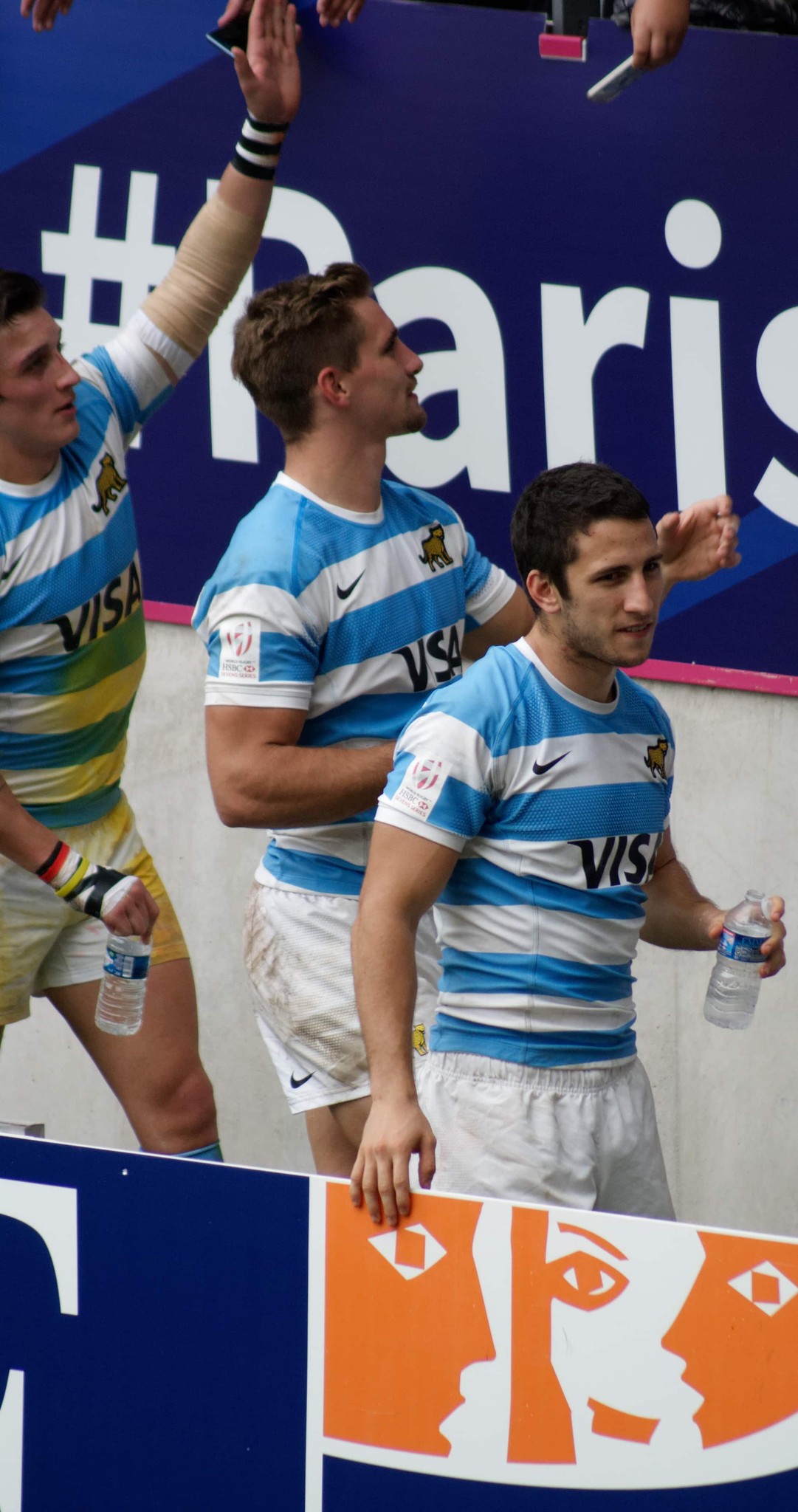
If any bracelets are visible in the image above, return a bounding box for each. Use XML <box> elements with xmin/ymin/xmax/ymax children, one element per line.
<box><xmin>232</xmin><ymin>115</ymin><xmax>288</xmax><ymax>181</ymax></box>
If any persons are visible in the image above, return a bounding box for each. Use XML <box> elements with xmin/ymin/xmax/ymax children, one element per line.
<box><xmin>191</xmin><ymin>265</ymin><xmax>738</xmax><ymax>1182</ymax></box>
<box><xmin>0</xmin><ymin>0</ymin><xmax>305</xmax><ymax>1171</ymax></box>
<box><xmin>11</xmin><ymin>0</ymin><xmax>798</xmax><ymax>71</ymax></box>
<box><xmin>351</xmin><ymin>463</ymin><xmax>786</xmax><ymax>1227</ymax></box>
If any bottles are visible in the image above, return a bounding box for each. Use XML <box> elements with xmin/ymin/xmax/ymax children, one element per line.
<box><xmin>703</xmin><ymin>889</ymin><xmax>771</xmax><ymax>1030</ymax></box>
<box><xmin>93</xmin><ymin>932</ymin><xmax>151</xmax><ymax>1036</ymax></box>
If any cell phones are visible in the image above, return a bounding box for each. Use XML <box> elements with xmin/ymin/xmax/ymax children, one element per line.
<box><xmin>207</xmin><ymin>13</ymin><xmax>252</xmax><ymax>58</ymax></box>
<box><xmin>588</xmin><ymin>55</ymin><xmax>642</xmax><ymax>104</ymax></box>
